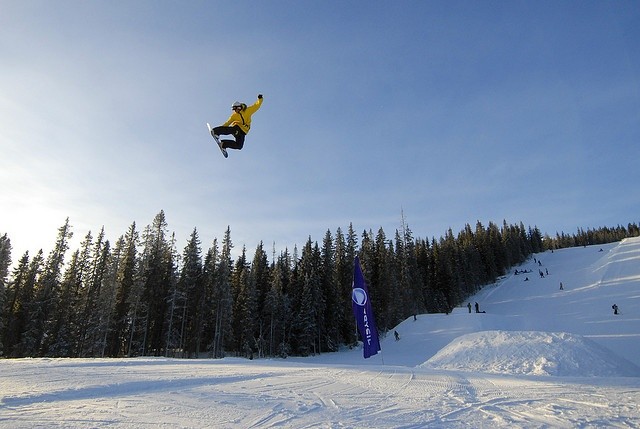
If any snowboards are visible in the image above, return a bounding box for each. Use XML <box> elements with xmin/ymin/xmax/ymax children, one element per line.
<box><xmin>207</xmin><ymin>121</ymin><xmax>229</xmax><ymax>159</ymax></box>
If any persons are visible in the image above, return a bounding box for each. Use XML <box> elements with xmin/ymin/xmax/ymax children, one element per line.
<box><xmin>474</xmin><ymin>302</ymin><xmax>479</xmax><ymax>313</ymax></box>
<box><xmin>394</xmin><ymin>331</ymin><xmax>399</xmax><ymax>340</ymax></box>
<box><xmin>612</xmin><ymin>304</ymin><xmax>618</xmax><ymax>313</ymax></box>
<box><xmin>212</xmin><ymin>94</ymin><xmax>263</xmax><ymax>150</ymax></box>
<box><xmin>514</xmin><ymin>269</ymin><xmax>528</xmax><ymax>280</ymax></box>
<box><xmin>560</xmin><ymin>282</ymin><xmax>563</xmax><ymax>289</ymax></box>
<box><xmin>534</xmin><ymin>258</ymin><xmax>548</xmax><ymax>277</ymax></box>
<box><xmin>583</xmin><ymin>244</ymin><xmax>587</xmax><ymax>248</ymax></box>
<box><xmin>467</xmin><ymin>303</ymin><xmax>471</xmax><ymax>313</ymax></box>
<box><xmin>600</xmin><ymin>248</ymin><xmax>602</xmax><ymax>251</ymax></box>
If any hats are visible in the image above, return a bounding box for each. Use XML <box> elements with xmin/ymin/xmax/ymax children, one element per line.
<box><xmin>232</xmin><ymin>102</ymin><xmax>241</xmax><ymax>109</ymax></box>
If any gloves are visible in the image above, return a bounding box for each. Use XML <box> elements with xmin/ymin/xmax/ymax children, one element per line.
<box><xmin>258</xmin><ymin>94</ymin><xmax>263</xmax><ymax>98</ymax></box>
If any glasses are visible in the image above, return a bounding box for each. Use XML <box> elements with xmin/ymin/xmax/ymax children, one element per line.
<box><xmin>232</xmin><ymin>106</ymin><xmax>241</xmax><ymax>110</ymax></box>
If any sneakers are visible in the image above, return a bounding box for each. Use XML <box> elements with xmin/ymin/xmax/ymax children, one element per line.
<box><xmin>220</xmin><ymin>141</ymin><xmax>226</xmax><ymax>152</ymax></box>
<box><xmin>211</xmin><ymin>128</ymin><xmax>219</xmax><ymax>139</ymax></box>
